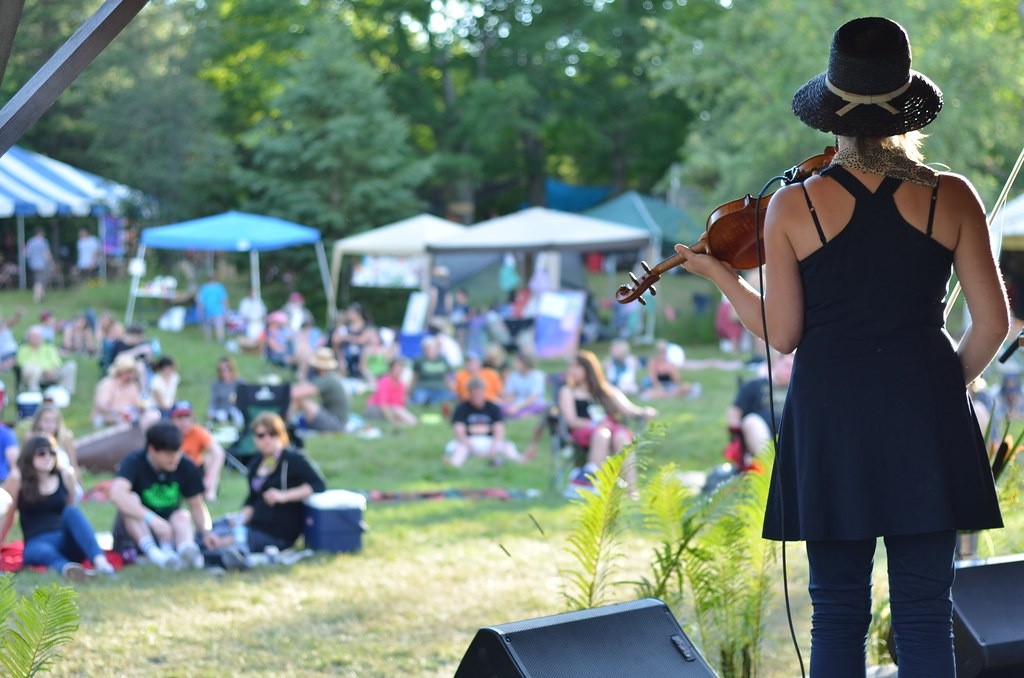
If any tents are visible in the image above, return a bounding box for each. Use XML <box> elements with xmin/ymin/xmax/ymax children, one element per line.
<box><xmin>322</xmin><ymin>213</ymin><xmax>472</xmax><ymax>340</ymax></box>
<box><xmin>582</xmin><ymin>191</ymin><xmax>703</xmax><ymax>341</ymax></box>
<box><xmin>122</xmin><ymin>209</ymin><xmax>344</xmax><ymax>356</ymax></box>
<box><xmin>0</xmin><ymin>145</ymin><xmax>144</xmax><ymax>290</ymax></box>
<box><xmin>428</xmin><ymin>204</ymin><xmax>659</xmax><ymax>345</ymax></box>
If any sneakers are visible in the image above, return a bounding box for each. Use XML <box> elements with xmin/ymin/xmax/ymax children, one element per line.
<box><xmin>222</xmin><ymin>548</ymin><xmax>248</xmax><ymax>571</ymax></box>
<box><xmin>572</xmin><ymin>473</ymin><xmax>596</xmax><ymax>487</ymax></box>
<box><xmin>163</xmin><ymin>554</ymin><xmax>179</xmax><ymax>571</ymax></box>
<box><xmin>63</xmin><ymin>562</ymin><xmax>95</xmax><ymax>583</ymax></box>
<box><xmin>180</xmin><ymin>542</ymin><xmax>204</xmax><ymax>570</ymax></box>
<box><xmin>96</xmin><ymin>563</ymin><xmax>115</xmax><ymax>578</ymax></box>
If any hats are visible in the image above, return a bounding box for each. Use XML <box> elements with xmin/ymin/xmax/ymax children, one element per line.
<box><xmin>173</xmin><ymin>400</ymin><xmax>193</xmax><ymax>417</ymax></box>
<box><xmin>792</xmin><ymin>17</ymin><xmax>943</xmax><ymax>138</ymax></box>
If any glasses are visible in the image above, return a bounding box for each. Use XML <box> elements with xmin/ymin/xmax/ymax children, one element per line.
<box><xmin>252</xmin><ymin>430</ymin><xmax>279</xmax><ymax>437</ymax></box>
<box><xmin>33</xmin><ymin>448</ymin><xmax>56</xmax><ymax>456</ymax></box>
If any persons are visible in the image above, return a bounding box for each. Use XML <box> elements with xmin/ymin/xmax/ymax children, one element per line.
<box><xmin>0</xmin><ymin>433</ymin><xmax>115</xmax><ymax>581</ymax></box>
<box><xmin>555</xmin><ymin>351</ymin><xmax>658</xmax><ymax>506</ymax></box>
<box><xmin>675</xmin><ymin>16</ymin><xmax>1014</xmax><ymax>678</ymax></box>
<box><xmin>108</xmin><ymin>422</ymin><xmax>222</xmax><ymax>574</ymax></box>
<box><xmin>446</xmin><ymin>377</ymin><xmax>526</xmax><ymax>470</ymax></box>
<box><xmin>200</xmin><ymin>414</ymin><xmax>327</xmax><ymax>566</ymax></box>
<box><xmin>0</xmin><ymin>219</ymin><xmax>807</xmax><ymax>453</ymax></box>
<box><xmin>953</xmin><ymin>227</ymin><xmax>1023</xmax><ymax>563</ymax></box>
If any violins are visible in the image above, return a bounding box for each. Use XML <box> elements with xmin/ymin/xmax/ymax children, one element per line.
<box><xmin>615</xmin><ymin>145</ymin><xmax>838</xmax><ymax>309</ymax></box>
